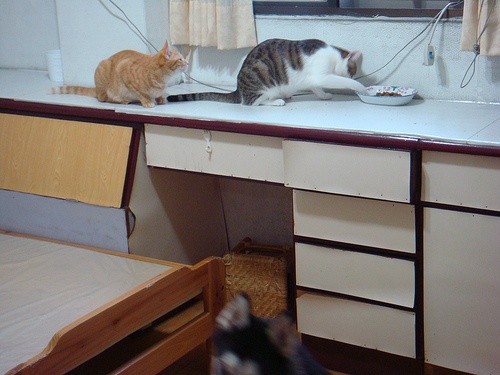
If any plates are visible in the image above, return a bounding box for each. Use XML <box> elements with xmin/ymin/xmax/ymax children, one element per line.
<box><xmin>355</xmin><ymin>85</ymin><xmax>418</xmax><ymax>106</ymax></box>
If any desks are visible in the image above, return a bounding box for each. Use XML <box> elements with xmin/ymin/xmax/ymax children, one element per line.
<box><xmin>0</xmin><ymin>95</ymin><xmax>500</xmax><ymax>375</ymax></box>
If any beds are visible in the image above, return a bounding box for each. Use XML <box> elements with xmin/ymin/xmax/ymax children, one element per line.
<box><xmin>0</xmin><ymin>232</ymin><xmax>225</xmax><ymax>375</ymax></box>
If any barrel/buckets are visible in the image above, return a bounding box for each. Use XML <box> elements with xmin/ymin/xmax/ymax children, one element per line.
<box><xmin>45</xmin><ymin>49</ymin><xmax>63</xmax><ymax>82</ymax></box>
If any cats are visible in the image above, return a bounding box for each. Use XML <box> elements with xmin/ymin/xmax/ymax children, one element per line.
<box><xmin>47</xmin><ymin>40</ymin><xmax>189</xmax><ymax>108</ymax></box>
<box><xmin>166</xmin><ymin>38</ymin><xmax>369</xmax><ymax>106</ymax></box>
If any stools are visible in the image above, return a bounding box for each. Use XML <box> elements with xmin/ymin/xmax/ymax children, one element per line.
<box><xmin>223</xmin><ymin>237</ymin><xmax>289</xmax><ymax>319</ymax></box>
